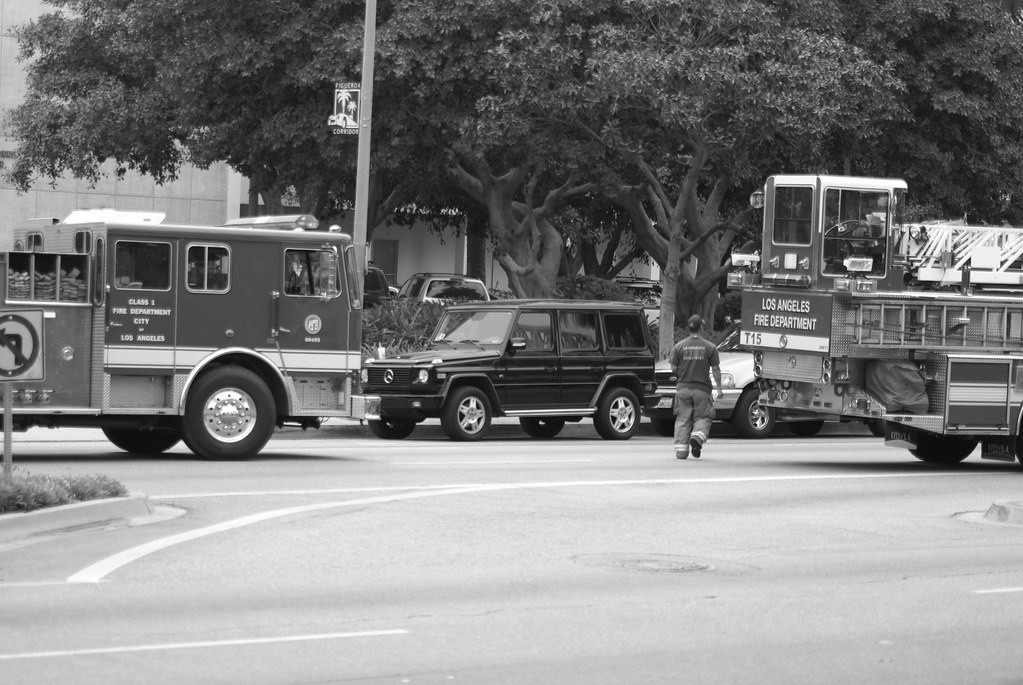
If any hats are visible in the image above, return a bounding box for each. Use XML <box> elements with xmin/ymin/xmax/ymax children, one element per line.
<box><xmin>688</xmin><ymin>315</ymin><xmax>706</xmax><ymax>328</ymax></box>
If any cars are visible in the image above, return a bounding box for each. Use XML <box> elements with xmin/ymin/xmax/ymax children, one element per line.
<box><xmin>641</xmin><ymin>329</ymin><xmax>822</xmax><ymax>436</ymax></box>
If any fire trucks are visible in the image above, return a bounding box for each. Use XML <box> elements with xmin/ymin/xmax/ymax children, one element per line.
<box><xmin>0</xmin><ymin>208</ymin><xmax>381</xmax><ymax>462</ymax></box>
<box><xmin>725</xmin><ymin>174</ymin><xmax>1023</xmax><ymax>467</ymax></box>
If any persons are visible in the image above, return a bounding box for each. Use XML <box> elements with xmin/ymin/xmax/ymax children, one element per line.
<box><xmin>668</xmin><ymin>315</ymin><xmax>724</xmax><ymax>459</ymax></box>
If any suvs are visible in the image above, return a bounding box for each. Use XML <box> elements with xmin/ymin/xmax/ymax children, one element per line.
<box><xmin>359</xmin><ymin>298</ymin><xmax>662</xmax><ymax>442</ymax></box>
<box><xmin>614</xmin><ymin>278</ymin><xmax>675</xmax><ymax>331</ymax></box>
<box><xmin>315</xmin><ymin>260</ymin><xmax>504</xmax><ymax>330</ymax></box>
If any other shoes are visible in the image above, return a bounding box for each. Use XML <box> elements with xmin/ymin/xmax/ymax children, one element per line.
<box><xmin>690</xmin><ymin>439</ymin><xmax>700</xmax><ymax>458</ymax></box>
<box><xmin>676</xmin><ymin>451</ymin><xmax>689</xmax><ymax>459</ymax></box>
